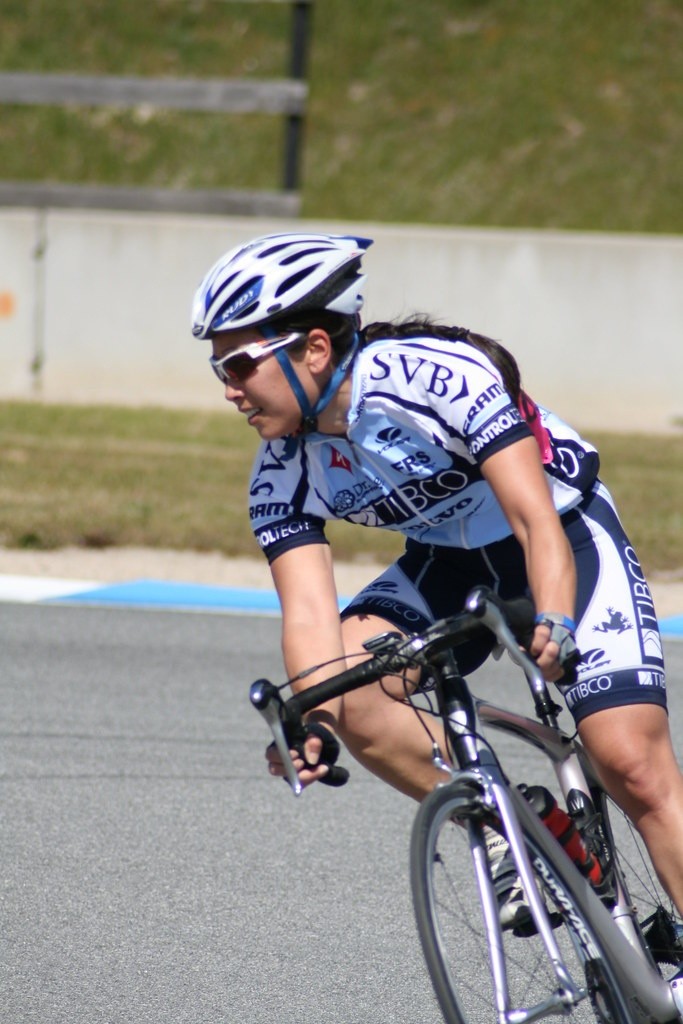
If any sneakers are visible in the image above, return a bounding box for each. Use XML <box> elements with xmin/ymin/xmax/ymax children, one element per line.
<box><xmin>484</xmin><ymin>832</ymin><xmax>548</xmax><ymax>932</ymax></box>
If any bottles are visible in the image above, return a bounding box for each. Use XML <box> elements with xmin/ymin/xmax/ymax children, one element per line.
<box><xmin>515</xmin><ymin>783</ymin><xmax>602</xmax><ymax>888</ymax></box>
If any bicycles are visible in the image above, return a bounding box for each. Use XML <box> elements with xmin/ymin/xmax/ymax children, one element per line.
<box><xmin>249</xmin><ymin>585</ymin><xmax>683</xmax><ymax>1024</ymax></box>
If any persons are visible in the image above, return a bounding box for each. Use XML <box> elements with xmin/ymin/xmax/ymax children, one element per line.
<box><xmin>189</xmin><ymin>232</ymin><xmax>683</xmax><ymax>933</ymax></box>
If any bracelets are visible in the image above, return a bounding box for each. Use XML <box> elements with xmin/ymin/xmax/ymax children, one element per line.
<box><xmin>533</xmin><ymin>612</ymin><xmax>578</xmax><ymax>633</ymax></box>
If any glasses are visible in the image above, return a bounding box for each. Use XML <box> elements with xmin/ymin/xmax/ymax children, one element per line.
<box><xmin>209</xmin><ymin>331</ymin><xmax>305</xmax><ymax>382</ymax></box>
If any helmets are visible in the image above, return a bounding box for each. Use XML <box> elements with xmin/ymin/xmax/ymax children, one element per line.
<box><xmin>192</xmin><ymin>231</ymin><xmax>374</xmax><ymax>340</ymax></box>
